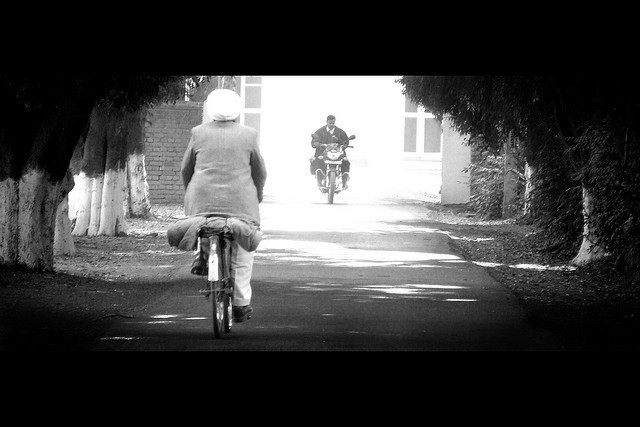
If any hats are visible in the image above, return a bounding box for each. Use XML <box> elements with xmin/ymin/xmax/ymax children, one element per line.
<box><xmin>206</xmin><ymin>88</ymin><xmax>243</xmax><ymax>122</ymax></box>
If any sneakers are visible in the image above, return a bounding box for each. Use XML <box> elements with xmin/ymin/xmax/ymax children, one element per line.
<box><xmin>232</xmin><ymin>304</ymin><xmax>253</xmax><ymax>323</ymax></box>
<box><xmin>190</xmin><ymin>252</ymin><xmax>208</xmax><ymax>274</ymax></box>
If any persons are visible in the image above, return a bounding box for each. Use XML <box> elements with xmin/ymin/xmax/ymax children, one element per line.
<box><xmin>310</xmin><ymin>114</ymin><xmax>351</xmax><ymax>190</ymax></box>
<box><xmin>181</xmin><ymin>85</ymin><xmax>266</xmax><ymax>324</ymax></box>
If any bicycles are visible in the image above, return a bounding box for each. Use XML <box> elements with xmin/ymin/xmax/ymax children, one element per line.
<box><xmin>194</xmin><ymin>212</ymin><xmax>251</xmax><ymax>339</ymax></box>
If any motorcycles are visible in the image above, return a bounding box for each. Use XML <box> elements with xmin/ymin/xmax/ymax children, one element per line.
<box><xmin>310</xmin><ymin>133</ymin><xmax>355</xmax><ymax>204</ymax></box>
